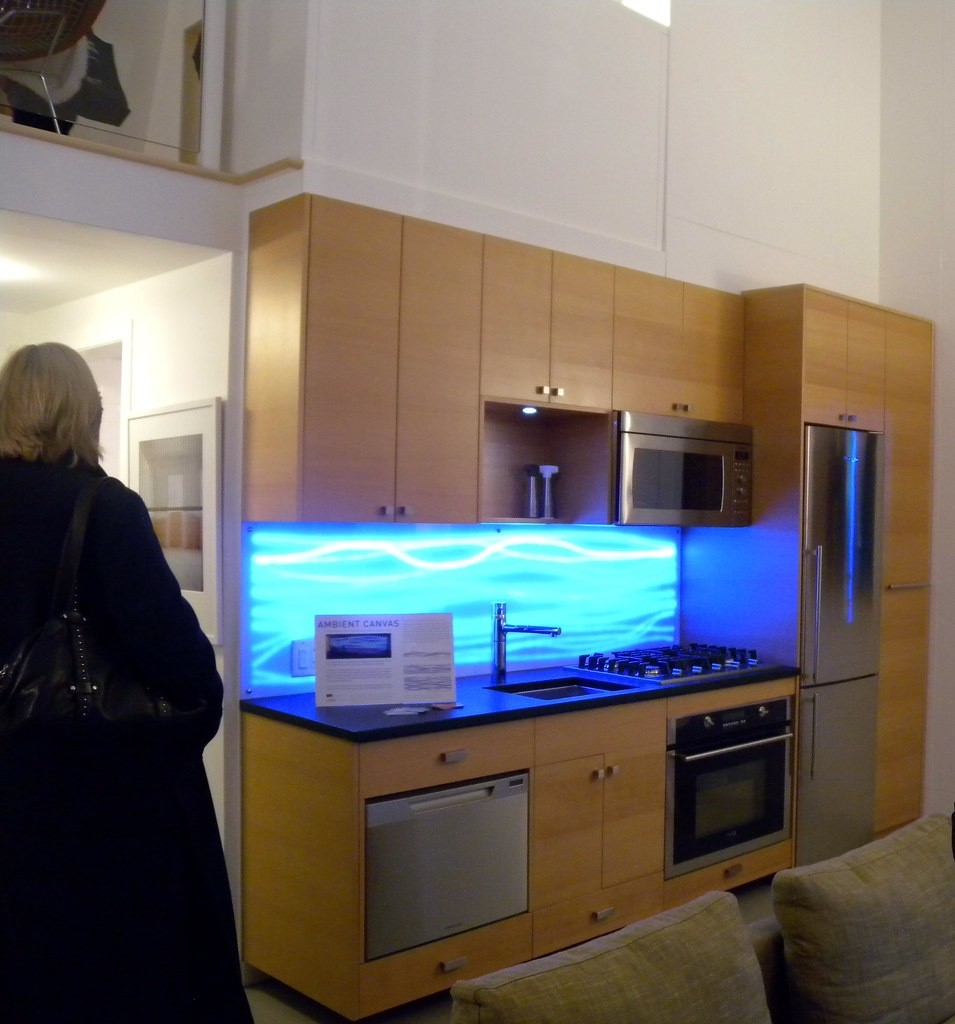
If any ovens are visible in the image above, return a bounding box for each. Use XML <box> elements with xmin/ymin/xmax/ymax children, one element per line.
<box><xmin>663</xmin><ymin>697</ymin><xmax>794</xmax><ymax>880</ymax></box>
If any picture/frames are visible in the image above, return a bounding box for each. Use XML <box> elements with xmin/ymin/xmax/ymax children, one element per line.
<box><xmin>124</xmin><ymin>396</ymin><xmax>224</xmax><ymax>644</ymax></box>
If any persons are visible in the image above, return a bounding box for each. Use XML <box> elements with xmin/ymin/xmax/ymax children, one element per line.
<box><xmin>0</xmin><ymin>341</ymin><xmax>256</xmax><ymax>1024</ymax></box>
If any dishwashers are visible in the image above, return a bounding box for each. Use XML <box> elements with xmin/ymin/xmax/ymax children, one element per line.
<box><xmin>363</xmin><ymin>772</ymin><xmax>529</xmax><ymax>961</ymax></box>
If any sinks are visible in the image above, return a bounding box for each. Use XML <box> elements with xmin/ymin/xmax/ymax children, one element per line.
<box><xmin>483</xmin><ymin>674</ymin><xmax>642</xmax><ymax>702</ymax></box>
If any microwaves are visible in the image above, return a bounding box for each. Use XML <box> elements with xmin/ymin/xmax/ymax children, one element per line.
<box><xmin>611</xmin><ymin>409</ymin><xmax>753</xmax><ymax>527</ymax></box>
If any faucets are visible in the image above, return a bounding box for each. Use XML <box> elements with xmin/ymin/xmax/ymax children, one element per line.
<box><xmin>492</xmin><ymin>602</ymin><xmax>562</xmax><ymax>684</ymax></box>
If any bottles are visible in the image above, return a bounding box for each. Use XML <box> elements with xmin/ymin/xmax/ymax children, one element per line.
<box><xmin>521</xmin><ymin>464</ymin><xmax>560</xmax><ymax>518</ymax></box>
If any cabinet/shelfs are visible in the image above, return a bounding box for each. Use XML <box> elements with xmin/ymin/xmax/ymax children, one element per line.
<box><xmin>736</xmin><ymin>283</ymin><xmax>883</xmax><ymax>431</ymax></box>
<box><xmin>481</xmin><ymin>234</ymin><xmax>612</xmax><ymax>412</ymax></box>
<box><xmin>878</xmin><ymin>309</ymin><xmax>937</xmax><ymax>832</ymax></box>
<box><xmin>611</xmin><ymin>265</ymin><xmax>745</xmax><ymax>425</ymax></box>
<box><xmin>239</xmin><ymin>678</ymin><xmax>801</xmax><ymax>1024</ymax></box>
<box><xmin>233</xmin><ymin>192</ymin><xmax>481</xmax><ymax>526</ymax></box>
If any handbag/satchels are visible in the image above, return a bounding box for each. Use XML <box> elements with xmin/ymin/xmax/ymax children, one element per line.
<box><xmin>0</xmin><ymin>478</ymin><xmax>172</xmax><ymax>743</ymax></box>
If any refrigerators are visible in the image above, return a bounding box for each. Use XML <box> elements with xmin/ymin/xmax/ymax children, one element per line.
<box><xmin>796</xmin><ymin>424</ymin><xmax>886</xmax><ymax>871</ymax></box>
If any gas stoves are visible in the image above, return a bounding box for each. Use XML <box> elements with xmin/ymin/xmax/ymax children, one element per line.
<box><xmin>566</xmin><ymin>642</ymin><xmax>786</xmax><ymax>685</ymax></box>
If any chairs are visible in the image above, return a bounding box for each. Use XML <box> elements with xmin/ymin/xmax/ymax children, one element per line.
<box><xmin>453</xmin><ymin>890</ymin><xmax>771</xmax><ymax>1024</ymax></box>
<box><xmin>770</xmin><ymin>809</ymin><xmax>955</xmax><ymax>1024</ymax></box>
